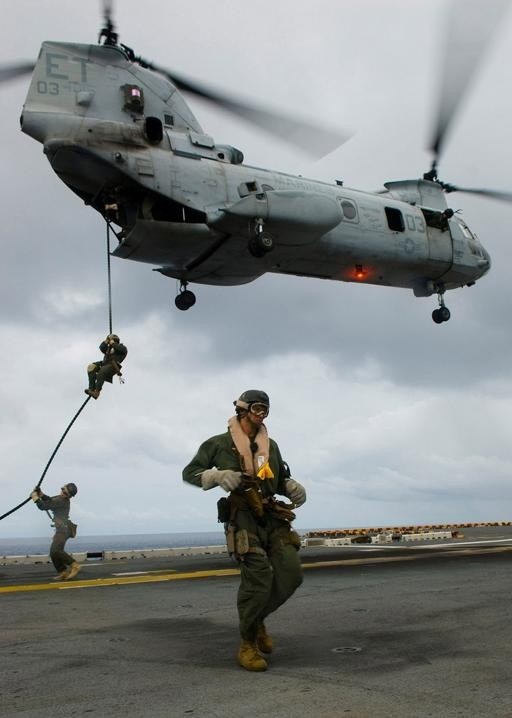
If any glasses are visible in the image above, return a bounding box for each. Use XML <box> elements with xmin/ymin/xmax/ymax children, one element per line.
<box><xmin>250</xmin><ymin>400</ymin><xmax>269</xmax><ymax>418</ymax></box>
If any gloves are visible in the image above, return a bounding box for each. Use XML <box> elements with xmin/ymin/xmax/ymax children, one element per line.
<box><xmin>282</xmin><ymin>479</ymin><xmax>306</xmax><ymax>508</ymax></box>
<box><xmin>202</xmin><ymin>466</ymin><xmax>242</xmax><ymax>492</ymax></box>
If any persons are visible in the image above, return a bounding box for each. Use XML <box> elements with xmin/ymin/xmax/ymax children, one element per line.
<box><xmin>83</xmin><ymin>331</ymin><xmax>128</xmax><ymax>399</ymax></box>
<box><xmin>30</xmin><ymin>482</ymin><xmax>81</xmax><ymax>581</ymax></box>
<box><xmin>427</xmin><ymin>209</ymin><xmax>454</xmax><ymax>232</ymax></box>
<box><xmin>133</xmin><ymin>181</ymin><xmax>155</xmax><ymax>222</ymax></box>
<box><xmin>180</xmin><ymin>387</ymin><xmax>307</xmax><ymax>672</ymax></box>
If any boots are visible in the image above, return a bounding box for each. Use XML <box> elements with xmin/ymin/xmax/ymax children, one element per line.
<box><xmin>67</xmin><ymin>562</ymin><xmax>82</xmax><ymax>579</ymax></box>
<box><xmin>257</xmin><ymin>632</ymin><xmax>272</xmax><ymax>653</ymax></box>
<box><xmin>54</xmin><ymin>569</ymin><xmax>70</xmax><ymax>580</ymax></box>
<box><xmin>237</xmin><ymin>638</ymin><xmax>268</xmax><ymax>672</ymax></box>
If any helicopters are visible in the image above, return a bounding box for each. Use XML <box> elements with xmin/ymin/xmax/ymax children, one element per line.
<box><xmin>0</xmin><ymin>0</ymin><xmax>512</xmax><ymax>324</ymax></box>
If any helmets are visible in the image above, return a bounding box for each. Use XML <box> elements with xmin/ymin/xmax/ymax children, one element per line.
<box><xmin>61</xmin><ymin>483</ymin><xmax>77</xmax><ymax>498</ymax></box>
<box><xmin>236</xmin><ymin>390</ymin><xmax>270</xmax><ymax>412</ymax></box>
